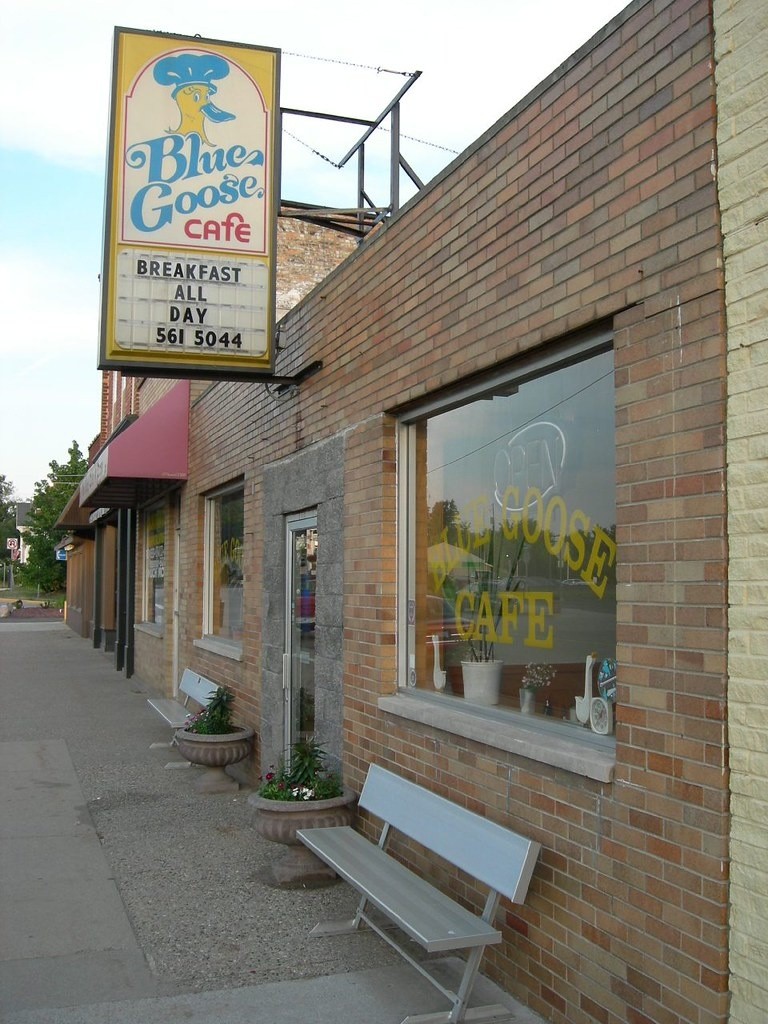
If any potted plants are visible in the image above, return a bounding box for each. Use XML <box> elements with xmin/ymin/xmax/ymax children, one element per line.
<box><xmin>445</xmin><ymin>501</ymin><xmax>526</xmax><ymax>705</ymax></box>
<box><xmin>219</xmin><ymin>539</ymin><xmax>244</xmax><ymax>637</ymax></box>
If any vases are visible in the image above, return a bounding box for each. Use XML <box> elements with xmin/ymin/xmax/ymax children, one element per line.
<box><xmin>176</xmin><ymin>722</ymin><xmax>255</xmax><ymax>792</ymax></box>
<box><xmin>300</xmin><ymin>575</ymin><xmax>316</xmax><ymax>596</ymax></box>
<box><xmin>519</xmin><ymin>688</ymin><xmax>536</xmax><ymax>715</ymax></box>
<box><xmin>248</xmin><ymin>787</ymin><xmax>355</xmax><ymax>888</ymax></box>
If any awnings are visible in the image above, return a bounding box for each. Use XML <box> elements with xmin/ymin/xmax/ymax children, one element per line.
<box><xmin>79</xmin><ymin>380</ymin><xmax>190</xmax><ymax>508</ymax></box>
<box><xmin>52</xmin><ymin>484</ymin><xmax>90</xmax><ymax>530</ymax></box>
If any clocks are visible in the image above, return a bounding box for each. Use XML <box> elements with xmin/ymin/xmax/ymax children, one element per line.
<box><xmin>590</xmin><ymin>697</ymin><xmax>613</xmax><ymax>736</ymax></box>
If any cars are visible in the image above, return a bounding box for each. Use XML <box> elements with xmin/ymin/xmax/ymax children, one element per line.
<box><xmin>425</xmin><ymin>568</ymin><xmax>597</xmax><ymax>652</ymax></box>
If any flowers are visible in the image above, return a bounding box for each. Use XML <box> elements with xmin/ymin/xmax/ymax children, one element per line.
<box><xmin>258</xmin><ymin>741</ymin><xmax>342</xmax><ymax>801</ymax></box>
<box><xmin>184</xmin><ymin>686</ymin><xmax>235</xmax><ymax>735</ymax></box>
<box><xmin>523</xmin><ymin>662</ymin><xmax>557</xmax><ymax>689</ymax></box>
<box><xmin>299</xmin><ymin>555</ymin><xmax>318</xmax><ymax>578</ymax></box>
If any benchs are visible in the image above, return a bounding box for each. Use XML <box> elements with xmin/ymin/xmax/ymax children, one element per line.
<box><xmin>147</xmin><ymin>666</ymin><xmax>225</xmax><ymax>746</ymax></box>
<box><xmin>294</xmin><ymin>761</ymin><xmax>541</xmax><ymax>1024</ymax></box>
